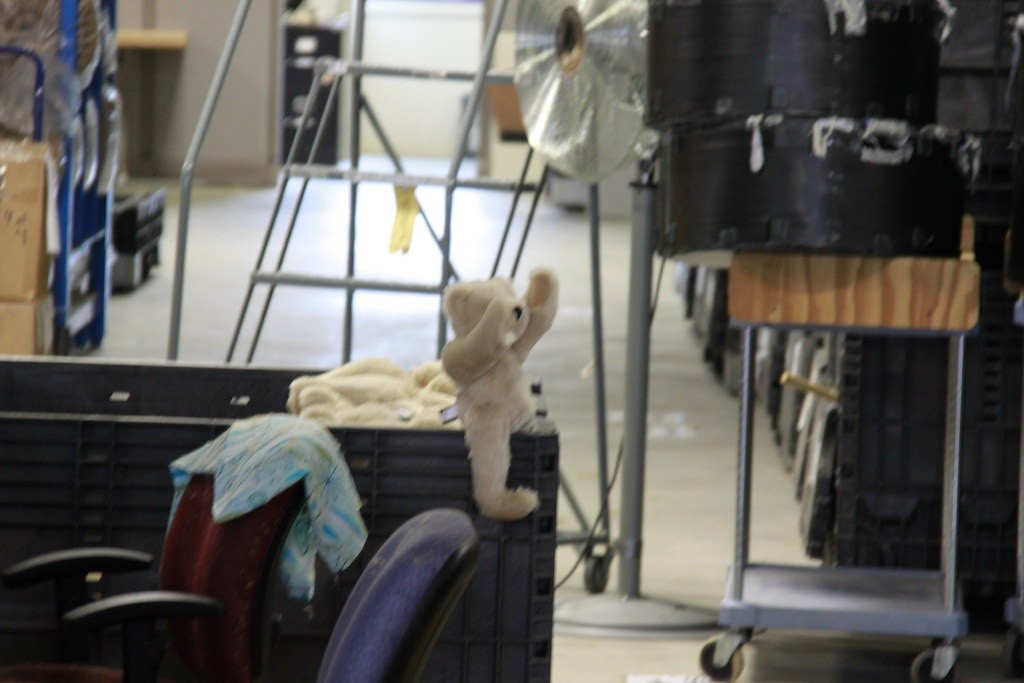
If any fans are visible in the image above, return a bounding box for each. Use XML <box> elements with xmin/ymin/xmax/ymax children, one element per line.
<box><xmin>511</xmin><ymin>0</ymin><xmax>645</xmax><ymax>185</ymax></box>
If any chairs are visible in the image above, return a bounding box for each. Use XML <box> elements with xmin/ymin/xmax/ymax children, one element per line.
<box><xmin>0</xmin><ymin>411</ymin><xmax>305</xmax><ymax>683</ymax></box>
<box><xmin>312</xmin><ymin>505</ymin><xmax>479</xmax><ymax>683</ymax></box>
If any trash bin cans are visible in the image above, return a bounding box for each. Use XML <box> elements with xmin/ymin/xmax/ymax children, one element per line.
<box><xmin>283</xmin><ymin>20</ymin><xmax>343</xmax><ymax>167</ymax></box>
<box><xmin>110</xmin><ymin>186</ymin><xmax>167</xmax><ymax>293</ymax></box>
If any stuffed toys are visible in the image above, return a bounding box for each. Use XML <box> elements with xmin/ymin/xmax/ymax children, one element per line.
<box><xmin>442</xmin><ymin>268</ymin><xmax>558</xmax><ymax>517</ymax></box>
<box><xmin>286</xmin><ymin>358</ymin><xmax>460</xmax><ymax>429</ymax></box>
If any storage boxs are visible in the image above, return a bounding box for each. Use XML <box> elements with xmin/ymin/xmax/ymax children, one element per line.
<box><xmin>0</xmin><ymin>355</ymin><xmax>562</xmax><ymax>682</ymax></box>
<box><xmin>0</xmin><ymin>138</ymin><xmax>66</xmax><ymax>303</ymax></box>
<box><xmin>0</xmin><ymin>294</ymin><xmax>54</xmax><ymax>360</ymax></box>
<box><xmin>653</xmin><ymin>110</ymin><xmax>964</xmax><ymax>258</ymax></box>
<box><xmin>646</xmin><ymin>0</ymin><xmax>944</xmax><ymax>131</ymax></box>
